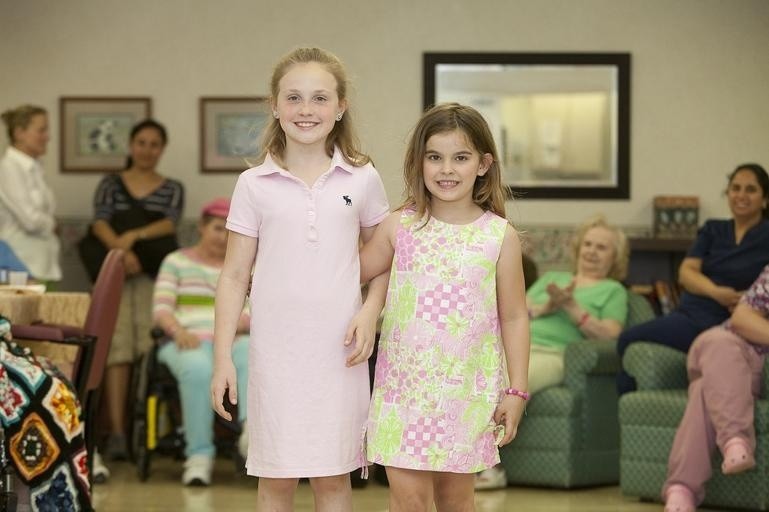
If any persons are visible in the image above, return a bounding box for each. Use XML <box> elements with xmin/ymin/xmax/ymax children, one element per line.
<box><xmin>0</xmin><ymin>316</ymin><xmax>95</xmax><ymax>510</ymax></box>
<box><xmin>361</xmin><ymin>101</ymin><xmax>531</xmax><ymax>511</ymax></box>
<box><xmin>91</xmin><ymin>120</ymin><xmax>184</xmax><ymax>462</ymax></box>
<box><xmin>86</xmin><ymin>445</ymin><xmax>110</xmax><ymax>486</ymax></box>
<box><xmin>614</xmin><ymin>163</ymin><xmax>769</xmax><ymax>392</ymax></box>
<box><xmin>152</xmin><ymin>199</ymin><xmax>249</xmax><ymax>489</ymax></box>
<box><xmin>662</xmin><ymin>267</ymin><xmax>767</xmax><ymax>511</ymax></box>
<box><xmin>210</xmin><ymin>49</ymin><xmax>391</xmax><ymax>510</ymax></box>
<box><xmin>0</xmin><ymin>103</ymin><xmax>62</xmax><ymax>283</ymax></box>
<box><xmin>472</xmin><ymin>217</ymin><xmax>628</xmax><ymax>491</ymax></box>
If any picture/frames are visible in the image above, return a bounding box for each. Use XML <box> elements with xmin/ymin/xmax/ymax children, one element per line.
<box><xmin>200</xmin><ymin>97</ymin><xmax>268</xmax><ymax>172</ymax></box>
<box><xmin>423</xmin><ymin>52</ymin><xmax>631</xmax><ymax>199</ymax></box>
<box><xmin>60</xmin><ymin>96</ymin><xmax>152</xmax><ymax>172</ymax></box>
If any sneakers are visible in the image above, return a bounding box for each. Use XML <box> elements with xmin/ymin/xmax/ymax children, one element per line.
<box><xmin>105</xmin><ymin>434</ymin><xmax>129</xmax><ymax>463</ymax></box>
<box><xmin>93</xmin><ymin>447</ymin><xmax>110</xmax><ymax>483</ymax></box>
<box><xmin>180</xmin><ymin>454</ymin><xmax>215</xmax><ymax>486</ymax></box>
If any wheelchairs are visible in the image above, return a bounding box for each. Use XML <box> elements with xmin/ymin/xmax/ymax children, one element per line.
<box><xmin>127</xmin><ymin>324</ymin><xmax>247</xmax><ymax>482</ymax></box>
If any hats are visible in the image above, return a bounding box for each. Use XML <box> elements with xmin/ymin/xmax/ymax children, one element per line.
<box><xmin>202</xmin><ymin>198</ymin><xmax>231</xmax><ymax>218</ymax></box>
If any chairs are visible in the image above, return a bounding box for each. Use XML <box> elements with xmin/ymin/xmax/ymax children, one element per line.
<box><xmin>10</xmin><ymin>249</ymin><xmax>127</xmax><ymax>493</ymax></box>
<box><xmin>499</xmin><ymin>292</ymin><xmax>657</xmax><ymax>489</ymax></box>
<box><xmin>617</xmin><ymin>342</ymin><xmax>769</xmax><ymax>512</ymax></box>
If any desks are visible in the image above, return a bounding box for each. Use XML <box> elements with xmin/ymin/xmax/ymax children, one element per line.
<box><xmin>0</xmin><ymin>291</ymin><xmax>90</xmax><ymax>362</ymax></box>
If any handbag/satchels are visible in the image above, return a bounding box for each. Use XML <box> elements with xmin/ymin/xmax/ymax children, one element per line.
<box><xmin>77</xmin><ymin>212</ymin><xmax>167</xmax><ymax>285</ymax></box>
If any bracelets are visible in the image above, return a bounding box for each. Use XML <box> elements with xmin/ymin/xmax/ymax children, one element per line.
<box><xmin>575</xmin><ymin>311</ymin><xmax>590</xmax><ymax>329</ymax></box>
<box><xmin>504</xmin><ymin>387</ymin><xmax>532</xmax><ymax>400</ymax></box>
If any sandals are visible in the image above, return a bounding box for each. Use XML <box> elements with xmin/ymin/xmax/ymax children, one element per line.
<box><xmin>721</xmin><ymin>436</ymin><xmax>756</xmax><ymax>474</ymax></box>
<box><xmin>474</xmin><ymin>467</ymin><xmax>507</xmax><ymax>491</ymax></box>
<box><xmin>664</xmin><ymin>484</ymin><xmax>696</xmax><ymax>512</ymax></box>
